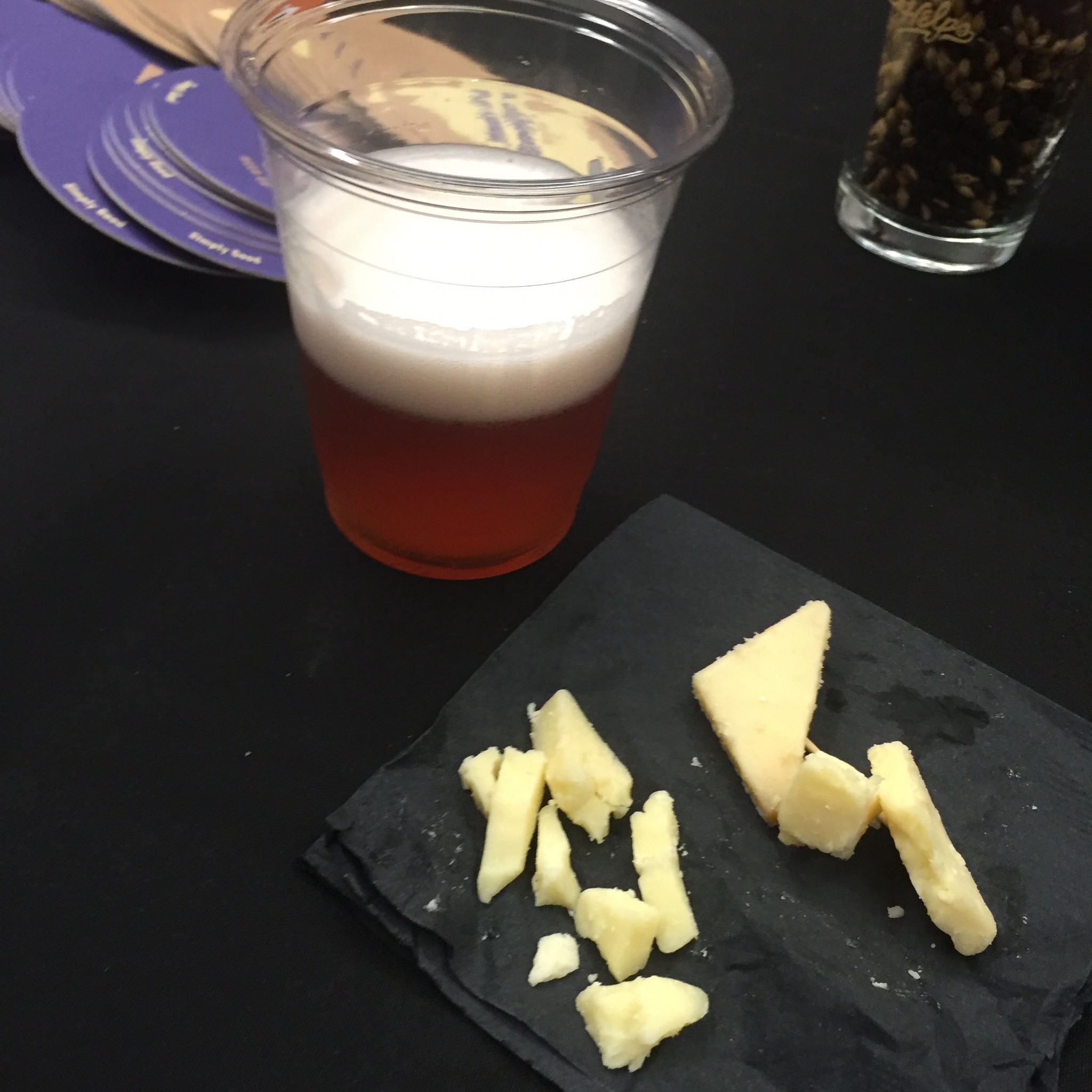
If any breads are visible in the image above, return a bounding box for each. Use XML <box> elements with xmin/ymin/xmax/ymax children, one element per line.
<box><xmin>691</xmin><ymin>600</ymin><xmax>997</xmax><ymax>955</ymax></box>
<box><xmin>455</xmin><ymin>687</ymin><xmax>712</xmax><ymax>1072</ymax></box>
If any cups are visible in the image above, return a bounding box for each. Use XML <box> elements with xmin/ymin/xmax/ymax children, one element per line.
<box><xmin>829</xmin><ymin>1</ymin><xmax>1092</xmax><ymax>274</ymax></box>
<box><xmin>217</xmin><ymin>1</ymin><xmax>737</xmax><ymax>581</ymax></box>
